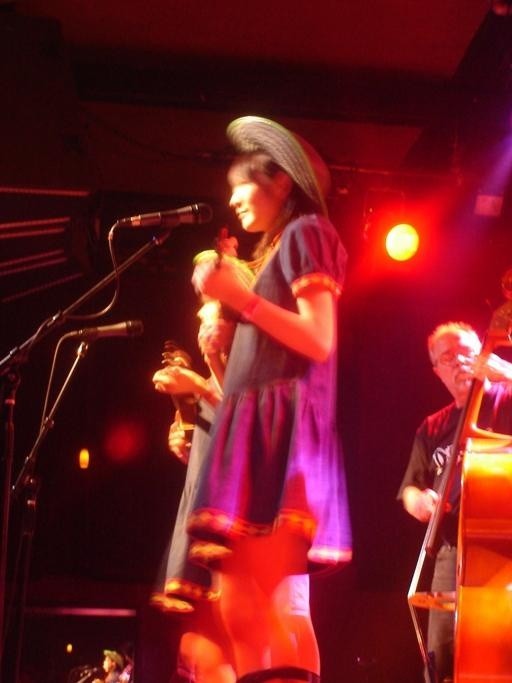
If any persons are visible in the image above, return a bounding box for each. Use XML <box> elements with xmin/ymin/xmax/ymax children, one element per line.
<box><xmin>395</xmin><ymin>320</ymin><xmax>512</xmax><ymax>683</ymax></box>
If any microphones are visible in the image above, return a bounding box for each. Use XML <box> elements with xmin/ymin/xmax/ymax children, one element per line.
<box><xmin>80</xmin><ymin>319</ymin><xmax>143</xmax><ymax>336</ymax></box>
<box><xmin>117</xmin><ymin>202</ymin><xmax>214</xmax><ymax>227</ymax></box>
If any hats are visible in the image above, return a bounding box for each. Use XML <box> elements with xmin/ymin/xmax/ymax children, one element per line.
<box><xmin>193</xmin><ymin>249</ymin><xmax>257</xmax><ymax>290</ymax></box>
<box><xmin>103</xmin><ymin>649</ymin><xmax>123</xmax><ymax>669</ymax></box>
<box><xmin>227</xmin><ymin>115</ymin><xmax>331</xmax><ymax>211</ymax></box>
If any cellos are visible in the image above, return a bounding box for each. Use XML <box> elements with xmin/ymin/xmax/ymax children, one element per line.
<box><xmin>451</xmin><ymin>267</ymin><xmax>512</xmax><ymax>683</ymax></box>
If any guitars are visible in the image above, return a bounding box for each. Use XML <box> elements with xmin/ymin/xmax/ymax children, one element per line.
<box><xmin>160</xmin><ymin>338</ymin><xmax>200</xmax><ymax>459</ymax></box>
